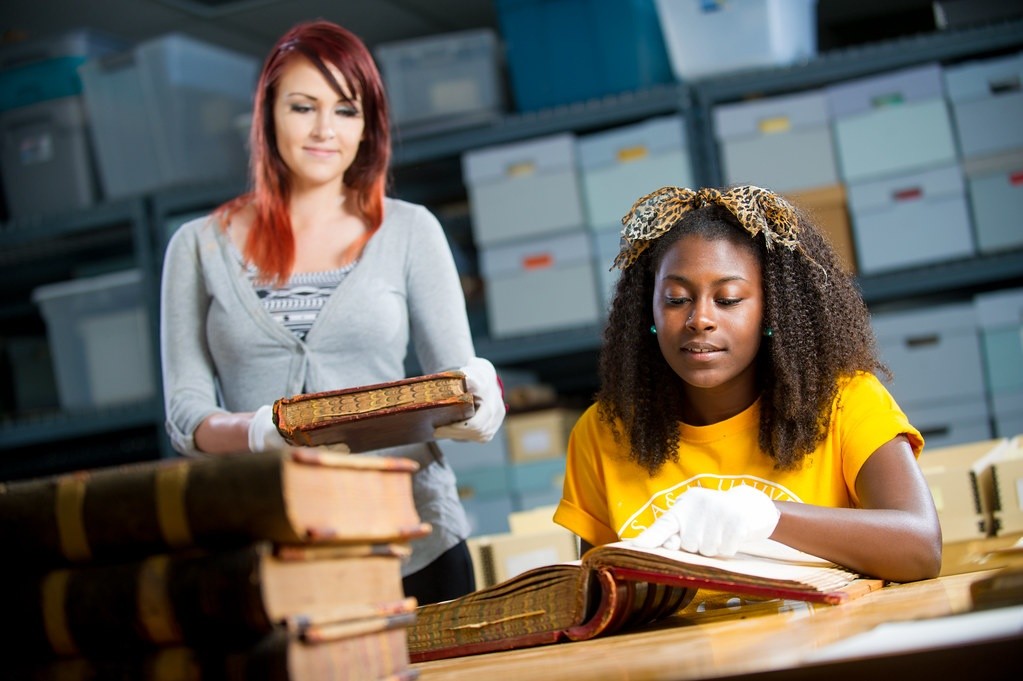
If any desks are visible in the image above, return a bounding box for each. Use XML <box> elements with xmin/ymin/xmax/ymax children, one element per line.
<box><xmin>408</xmin><ymin>566</ymin><xmax>1007</xmax><ymax>681</ymax></box>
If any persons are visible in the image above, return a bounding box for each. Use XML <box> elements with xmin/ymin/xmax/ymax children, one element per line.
<box><xmin>551</xmin><ymin>185</ymin><xmax>943</xmax><ymax>585</ymax></box>
<box><xmin>158</xmin><ymin>19</ymin><xmax>506</xmax><ymax>606</ymax></box>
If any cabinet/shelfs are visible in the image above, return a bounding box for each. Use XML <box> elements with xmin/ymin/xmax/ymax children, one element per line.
<box><xmin>0</xmin><ymin>25</ymin><xmax>1021</xmax><ymax>448</ymax></box>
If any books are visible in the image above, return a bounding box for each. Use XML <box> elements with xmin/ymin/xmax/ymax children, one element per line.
<box><xmin>0</xmin><ymin>613</ymin><xmax>420</xmax><ymax>681</ymax></box>
<box><xmin>401</xmin><ymin>541</ymin><xmax>886</xmax><ymax>664</ymax></box>
<box><xmin>273</xmin><ymin>369</ymin><xmax>480</xmax><ymax>454</ymax></box>
<box><xmin>0</xmin><ymin>445</ymin><xmax>433</xmax><ymax>548</ymax></box>
<box><xmin>0</xmin><ymin>541</ymin><xmax>419</xmax><ymax>659</ymax></box>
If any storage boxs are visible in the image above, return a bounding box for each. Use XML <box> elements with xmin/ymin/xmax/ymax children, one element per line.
<box><xmin>0</xmin><ymin>0</ymin><xmax>1023</xmax><ymax>590</ymax></box>
